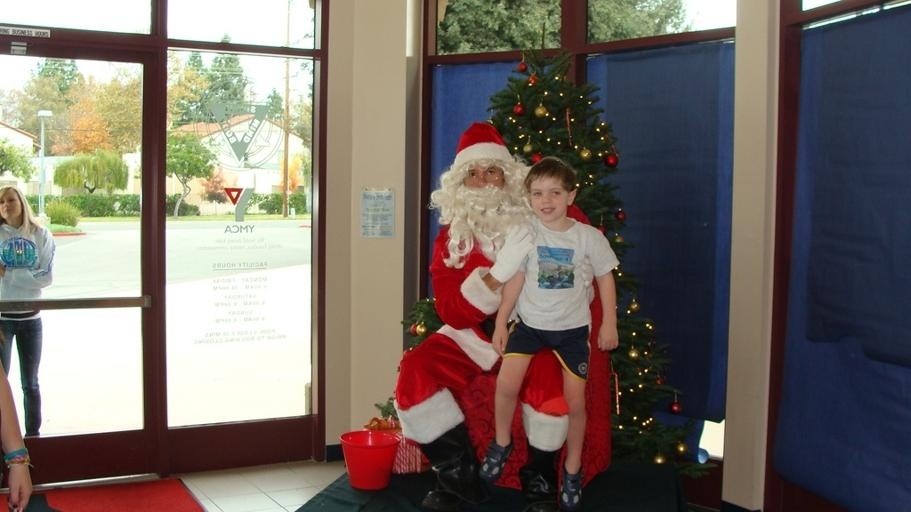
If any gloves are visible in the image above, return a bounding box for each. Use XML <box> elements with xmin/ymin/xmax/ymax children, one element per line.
<box><xmin>490</xmin><ymin>223</ymin><xmax>536</xmax><ymax>284</ymax></box>
<box><xmin>582</xmin><ymin>256</ymin><xmax>595</xmax><ymax>289</ymax></box>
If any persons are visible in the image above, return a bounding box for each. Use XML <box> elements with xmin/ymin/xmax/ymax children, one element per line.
<box><xmin>0</xmin><ymin>185</ymin><xmax>57</xmax><ymax>438</ymax></box>
<box><xmin>472</xmin><ymin>153</ymin><xmax>622</xmax><ymax>511</ymax></box>
<box><xmin>390</xmin><ymin>118</ymin><xmax>569</xmax><ymax>512</ymax></box>
<box><xmin>0</xmin><ymin>360</ymin><xmax>35</xmax><ymax>512</ymax></box>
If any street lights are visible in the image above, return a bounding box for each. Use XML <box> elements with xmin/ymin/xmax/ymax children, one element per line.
<box><xmin>38</xmin><ymin>110</ymin><xmax>53</xmax><ymax>217</ymax></box>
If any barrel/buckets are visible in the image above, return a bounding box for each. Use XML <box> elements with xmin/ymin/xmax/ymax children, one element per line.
<box><xmin>340</xmin><ymin>429</ymin><xmax>400</xmax><ymax>490</ymax></box>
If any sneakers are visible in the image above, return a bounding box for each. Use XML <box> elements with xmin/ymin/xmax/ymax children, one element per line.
<box><xmin>478</xmin><ymin>436</ymin><xmax>513</xmax><ymax>483</ymax></box>
<box><xmin>560</xmin><ymin>456</ymin><xmax>584</xmax><ymax>507</ymax></box>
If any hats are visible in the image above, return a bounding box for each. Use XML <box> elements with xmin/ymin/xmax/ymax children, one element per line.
<box><xmin>451</xmin><ymin>121</ymin><xmax>512</xmax><ymax>164</ymax></box>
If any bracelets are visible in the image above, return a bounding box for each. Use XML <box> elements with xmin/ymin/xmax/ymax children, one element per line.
<box><xmin>2</xmin><ymin>447</ymin><xmax>32</xmax><ymax>471</ymax></box>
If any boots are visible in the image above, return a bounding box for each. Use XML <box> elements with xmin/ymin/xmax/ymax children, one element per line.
<box><xmin>518</xmin><ymin>402</ymin><xmax>569</xmax><ymax>512</ymax></box>
<box><xmin>393</xmin><ymin>389</ymin><xmax>481</xmax><ymax>512</ymax></box>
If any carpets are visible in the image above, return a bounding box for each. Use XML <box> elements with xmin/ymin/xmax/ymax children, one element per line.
<box><xmin>296</xmin><ymin>461</ymin><xmax>687</xmax><ymax>512</ymax></box>
<box><xmin>0</xmin><ymin>477</ymin><xmax>205</xmax><ymax>512</ymax></box>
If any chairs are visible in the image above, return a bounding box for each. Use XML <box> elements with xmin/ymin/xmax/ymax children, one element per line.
<box><xmin>453</xmin><ymin>277</ymin><xmax>613</xmax><ymax>493</ymax></box>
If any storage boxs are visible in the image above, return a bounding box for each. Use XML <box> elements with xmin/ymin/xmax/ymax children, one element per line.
<box><xmin>363</xmin><ymin>427</ymin><xmax>434</xmax><ymax>474</ymax></box>
